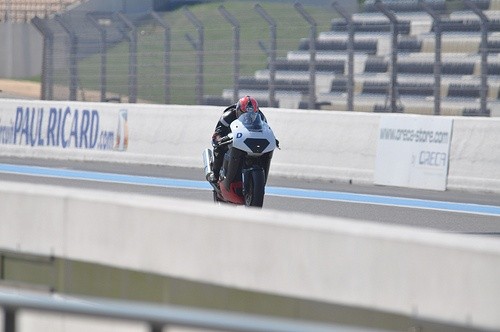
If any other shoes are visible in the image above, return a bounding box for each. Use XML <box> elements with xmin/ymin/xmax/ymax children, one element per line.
<box><xmin>210</xmin><ymin>172</ymin><xmax>218</xmax><ymax>182</ymax></box>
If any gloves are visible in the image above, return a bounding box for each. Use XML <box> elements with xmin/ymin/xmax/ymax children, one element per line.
<box><xmin>220</xmin><ymin>136</ymin><xmax>230</xmax><ymax>142</ymax></box>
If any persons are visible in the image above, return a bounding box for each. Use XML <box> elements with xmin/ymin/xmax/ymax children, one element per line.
<box><xmin>210</xmin><ymin>95</ymin><xmax>280</xmax><ymax>182</ymax></box>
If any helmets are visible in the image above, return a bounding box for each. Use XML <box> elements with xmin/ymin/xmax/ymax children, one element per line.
<box><xmin>235</xmin><ymin>96</ymin><xmax>259</xmax><ymax>124</ymax></box>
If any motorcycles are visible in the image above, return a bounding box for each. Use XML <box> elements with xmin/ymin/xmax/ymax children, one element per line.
<box><xmin>202</xmin><ymin>111</ymin><xmax>281</xmax><ymax>208</ymax></box>
